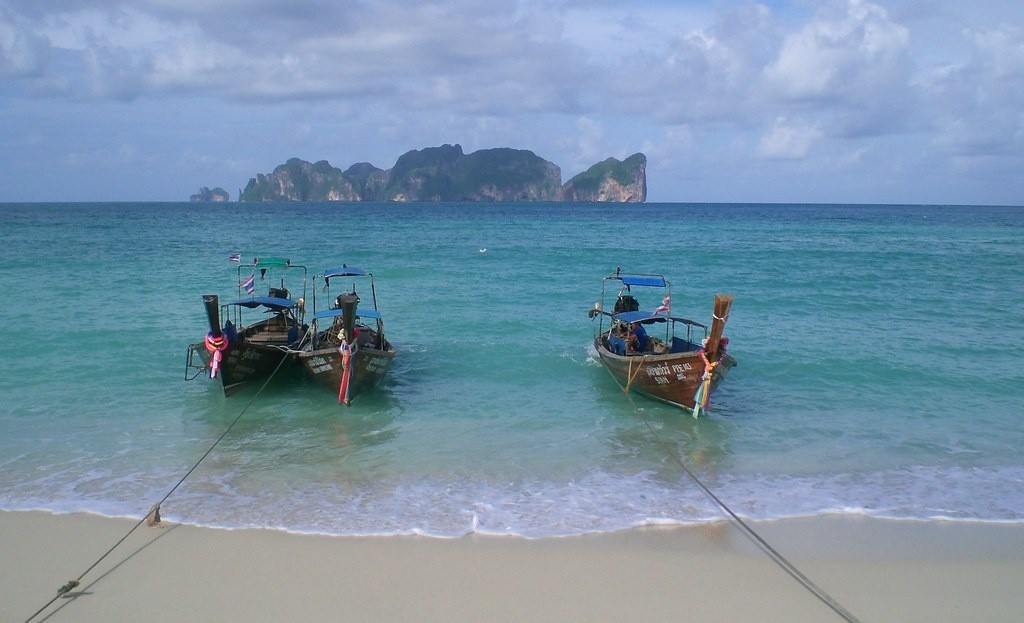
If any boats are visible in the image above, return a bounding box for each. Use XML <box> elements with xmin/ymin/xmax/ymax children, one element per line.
<box><xmin>183</xmin><ymin>257</ymin><xmax>309</xmax><ymax>399</ymax></box>
<box><xmin>297</xmin><ymin>263</ymin><xmax>397</xmax><ymax>406</ymax></box>
<box><xmin>587</xmin><ymin>266</ymin><xmax>737</xmax><ymax>413</ymax></box>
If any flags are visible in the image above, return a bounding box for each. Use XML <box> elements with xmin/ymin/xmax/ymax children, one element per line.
<box><xmin>228</xmin><ymin>252</ymin><xmax>241</xmax><ymax>264</ymax></box>
<box><xmin>650</xmin><ymin>292</ymin><xmax>670</xmax><ymax>318</ymax></box>
<box><xmin>238</xmin><ymin>273</ymin><xmax>254</xmax><ymax>296</ymax></box>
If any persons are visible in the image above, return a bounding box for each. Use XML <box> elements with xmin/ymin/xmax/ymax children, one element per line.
<box><xmin>620</xmin><ymin>321</ymin><xmax>649</xmax><ymax>352</ymax></box>
